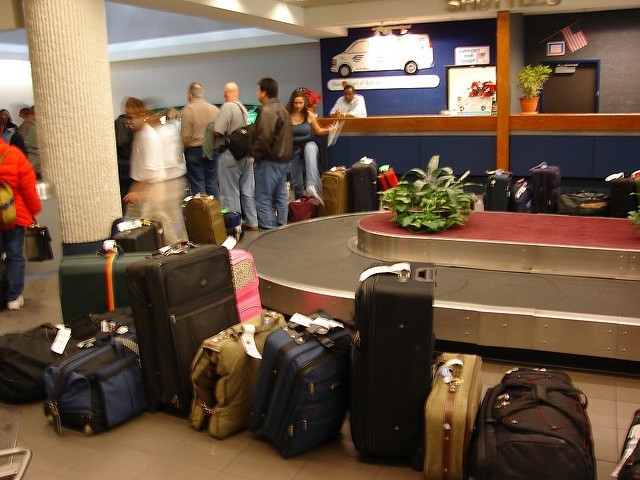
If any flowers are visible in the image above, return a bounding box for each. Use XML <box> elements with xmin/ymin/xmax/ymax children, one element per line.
<box><xmin>305</xmin><ymin>89</ymin><xmax>321</xmax><ymax>108</ymax></box>
<box><xmin>466</xmin><ymin>80</ymin><xmax>496</xmax><ymax>99</ymax></box>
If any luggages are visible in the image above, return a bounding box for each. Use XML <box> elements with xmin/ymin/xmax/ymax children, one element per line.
<box><xmin>351</xmin><ymin>157</ymin><xmax>379</xmax><ymax>212</ymax></box>
<box><xmin>605</xmin><ymin>171</ymin><xmax>640</xmax><ymax>218</ymax></box>
<box><xmin>126</xmin><ymin>239</ymin><xmax>240</xmax><ymax>420</ymax></box>
<box><xmin>349</xmin><ymin>261</ymin><xmax>436</xmax><ymax>471</ymax></box>
<box><xmin>59</xmin><ymin>238</ymin><xmax>152</xmax><ymax>325</ymax></box>
<box><xmin>483</xmin><ymin>170</ymin><xmax>512</xmax><ymax>212</ymax></box>
<box><xmin>110</xmin><ymin>218</ymin><xmax>165</xmax><ymax>251</ymax></box>
<box><xmin>530</xmin><ymin>162</ymin><xmax>560</xmax><ymax>213</ymax></box>
<box><xmin>186</xmin><ymin>193</ymin><xmax>229</xmax><ymax>247</ymax></box>
<box><xmin>378</xmin><ymin>165</ymin><xmax>399</xmax><ymax>191</ymax></box>
<box><xmin>227</xmin><ymin>250</ymin><xmax>262</xmax><ymax>325</ymax></box>
<box><xmin>560</xmin><ymin>190</ymin><xmax>609</xmax><ymax>217</ymax></box>
<box><xmin>321</xmin><ymin>166</ymin><xmax>350</xmax><ymax>214</ymax></box>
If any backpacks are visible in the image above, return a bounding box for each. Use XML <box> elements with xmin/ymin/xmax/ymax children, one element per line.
<box><xmin>1</xmin><ymin>181</ymin><xmax>17</xmax><ymax>224</ymax></box>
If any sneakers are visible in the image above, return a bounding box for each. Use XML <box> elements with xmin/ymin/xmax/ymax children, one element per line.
<box><xmin>8</xmin><ymin>296</ymin><xmax>24</xmax><ymax>310</ymax></box>
<box><xmin>247</xmin><ymin>227</ymin><xmax>259</xmax><ymax>230</ymax></box>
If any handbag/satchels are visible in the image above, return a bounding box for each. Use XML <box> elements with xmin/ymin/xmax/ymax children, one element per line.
<box><xmin>25</xmin><ymin>221</ymin><xmax>54</xmax><ymax>263</ymax></box>
<box><xmin>289</xmin><ymin>197</ymin><xmax>319</xmax><ymax>222</ymax></box>
<box><xmin>509</xmin><ymin>179</ymin><xmax>533</xmax><ymax>213</ymax></box>
<box><xmin>223</xmin><ymin>211</ymin><xmax>244</xmax><ymax>242</ymax></box>
<box><xmin>187</xmin><ymin>311</ymin><xmax>287</xmax><ymax>438</ymax></box>
<box><xmin>611</xmin><ymin>408</ymin><xmax>640</xmax><ymax>480</ymax></box>
<box><xmin>226</xmin><ymin>100</ymin><xmax>254</xmax><ymax>160</ymax></box>
<box><xmin>250</xmin><ymin>308</ymin><xmax>357</xmax><ymax>460</ymax></box>
<box><xmin>45</xmin><ymin>332</ymin><xmax>159</xmax><ymax>437</ymax></box>
<box><xmin>467</xmin><ymin>365</ymin><xmax>597</xmax><ymax>480</ymax></box>
<box><xmin>0</xmin><ymin>325</ymin><xmax>83</xmax><ymax>405</ymax></box>
<box><xmin>87</xmin><ymin>307</ymin><xmax>137</xmax><ymax>344</ymax></box>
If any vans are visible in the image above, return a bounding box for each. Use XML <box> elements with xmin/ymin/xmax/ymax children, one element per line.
<box><xmin>330</xmin><ymin>33</ymin><xmax>435</xmax><ymax>77</ymax></box>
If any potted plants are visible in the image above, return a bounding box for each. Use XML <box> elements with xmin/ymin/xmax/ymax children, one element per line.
<box><xmin>515</xmin><ymin>64</ymin><xmax>552</xmax><ymax>115</ymax></box>
<box><xmin>376</xmin><ymin>154</ymin><xmax>483</xmax><ymax>234</ymax></box>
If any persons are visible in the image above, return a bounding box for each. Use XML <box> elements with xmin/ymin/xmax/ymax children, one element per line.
<box><xmin>19</xmin><ymin>107</ymin><xmax>30</xmax><ymax>120</ymax></box>
<box><xmin>180</xmin><ymin>81</ymin><xmax>222</xmax><ymax>199</ymax></box>
<box><xmin>2</xmin><ymin>129</ymin><xmax>28</xmax><ymax>155</ymax></box>
<box><xmin>285</xmin><ymin>91</ymin><xmax>337</xmax><ymax>207</ymax></box>
<box><xmin>330</xmin><ymin>84</ymin><xmax>368</xmax><ymax>118</ymax></box>
<box><xmin>17</xmin><ymin>104</ymin><xmax>41</xmax><ymax>179</ymax></box>
<box><xmin>115</xmin><ymin>97</ymin><xmax>189</xmax><ymax>245</ymax></box>
<box><xmin>214</xmin><ymin>83</ymin><xmax>260</xmax><ymax>231</ymax></box>
<box><xmin>1</xmin><ymin>119</ymin><xmax>43</xmax><ymax>310</ymax></box>
<box><xmin>254</xmin><ymin>78</ymin><xmax>293</xmax><ymax>231</ymax></box>
<box><xmin>0</xmin><ymin>108</ymin><xmax>17</xmax><ymax>127</ymax></box>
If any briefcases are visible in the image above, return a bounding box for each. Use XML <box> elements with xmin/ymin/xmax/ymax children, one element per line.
<box><xmin>422</xmin><ymin>350</ymin><xmax>483</xmax><ymax>479</ymax></box>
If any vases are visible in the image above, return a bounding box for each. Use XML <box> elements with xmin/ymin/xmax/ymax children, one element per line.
<box><xmin>307</xmin><ymin>107</ymin><xmax>315</xmax><ymax>113</ymax></box>
